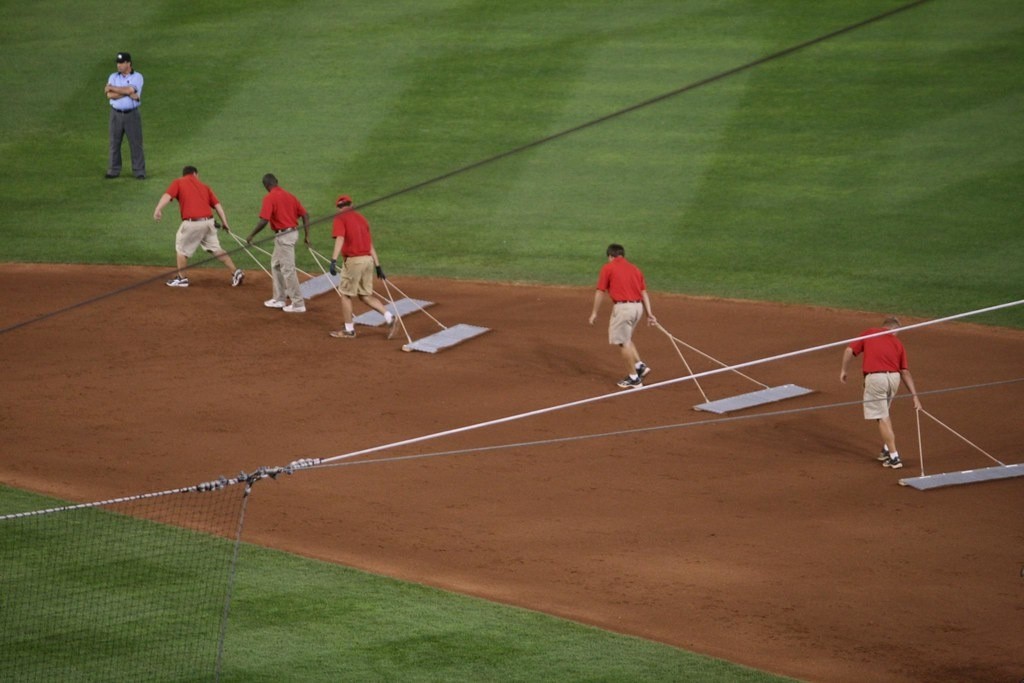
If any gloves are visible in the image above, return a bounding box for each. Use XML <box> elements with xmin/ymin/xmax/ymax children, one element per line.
<box><xmin>375</xmin><ymin>266</ymin><xmax>385</xmax><ymax>280</ymax></box>
<box><xmin>330</xmin><ymin>259</ymin><xmax>337</xmax><ymax>276</ymax></box>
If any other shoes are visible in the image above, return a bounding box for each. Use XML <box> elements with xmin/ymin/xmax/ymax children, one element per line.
<box><xmin>106</xmin><ymin>174</ymin><xmax>118</xmax><ymax>178</ymax></box>
<box><xmin>136</xmin><ymin>175</ymin><xmax>144</xmax><ymax>179</ymax></box>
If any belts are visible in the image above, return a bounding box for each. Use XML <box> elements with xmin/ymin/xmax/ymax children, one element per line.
<box><xmin>864</xmin><ymin>372</ymin><xmax>894</xmax><ymax>376</ymax></box>
<box><xmin>615</xmin><ymin>300</ymin><xmax>641</xmax><ymax>304</ymax></box>
<box><xmin>275</xmin><ymin>227</ymin><xmax>294</xmax><ymax>233</ymax></box>
<box><xmin>113</xmin><ymin>107</ymin><xmax>137</xmax><ymax>114</ymax></box>
<box><xmin>185</xmin><ymin>216</ymin><xmax>213</xmax><ymax>221</ymax></box>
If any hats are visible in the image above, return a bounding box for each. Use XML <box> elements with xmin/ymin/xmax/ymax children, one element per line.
<box><xmin>116</xmin><ymin>52</ymin><xmax>131</xmax><ymax>63</ymax></box>
<box><xmin>336</xmin><ymin>196</ymin><xmax>352</xmax><ymax>206</ymax></box>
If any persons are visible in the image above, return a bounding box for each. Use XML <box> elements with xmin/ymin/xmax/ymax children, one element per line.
<box><xmin>588</xmin><ymin>244</ymin><xmax>658</xmax><ymax>388</ymax></box>
<box><xmin>246</xmin><ymin>173</ymin><xmax>313</xmax><ymax>313</ymax></box>
<box><xmin>153</xmin><ymin>166</ymin><xmax>245</xmax><ymax>288</ymax></box>
<box><xmin>328</xmin><ymin>195</ymin><xmax>399</xmax><ymax>340</ymax></box>
<box><xmin>839</xmin><ymin>317</ymin><xmax>923</xmax><ymax>469</ymax></box>
<box><xmin>104</xmin><ymin>52</ymin><xmax>147</xmax><ymax>181</ymax></box>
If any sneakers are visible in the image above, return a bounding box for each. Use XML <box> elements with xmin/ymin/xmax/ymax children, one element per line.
<box><xmin>636</xmin><ymin>363</ymin><xmax>651</xmax><ymax>380</ymax></box>
<box><xmin>232</xmin><ymin>270</ymin><xmax>246</xmax><ymax>285</ymax></box>
<box><xmin>387</xmin><ymin>315</ymin><xmax>401</xmax><ymax>339</ymax></box>
<box><xmin>166</xmin><ymin>275</ymin><xmax>189</xmax><ymax>287</ymax></box>
<box><xmin>616</xmin><ymin>376</ymin><xmax>643</xmax><ymax>388</ymax></box>
<box><xmin>329</xmin><ymin>330</ymin><xmax>356</xmax><ymax>337</ymax></box>
<box><xmin>283</xmin><ymin>304</ymin><xmax>306</xmax><ymax>312</ymax></box>
<box><xmin>883</xmin><ymin>456</ymin><xmax>902</xmax><ymax>468</ymax></box>
<box><xmin>877</xmin><ymin>449</ymin><xmax>889</xmax><ymax>460</ymax></box>
<box><xmin>264</xmin><ymin>299</ymin><xmax>286</xmax><ymax>308</ymax></box>
